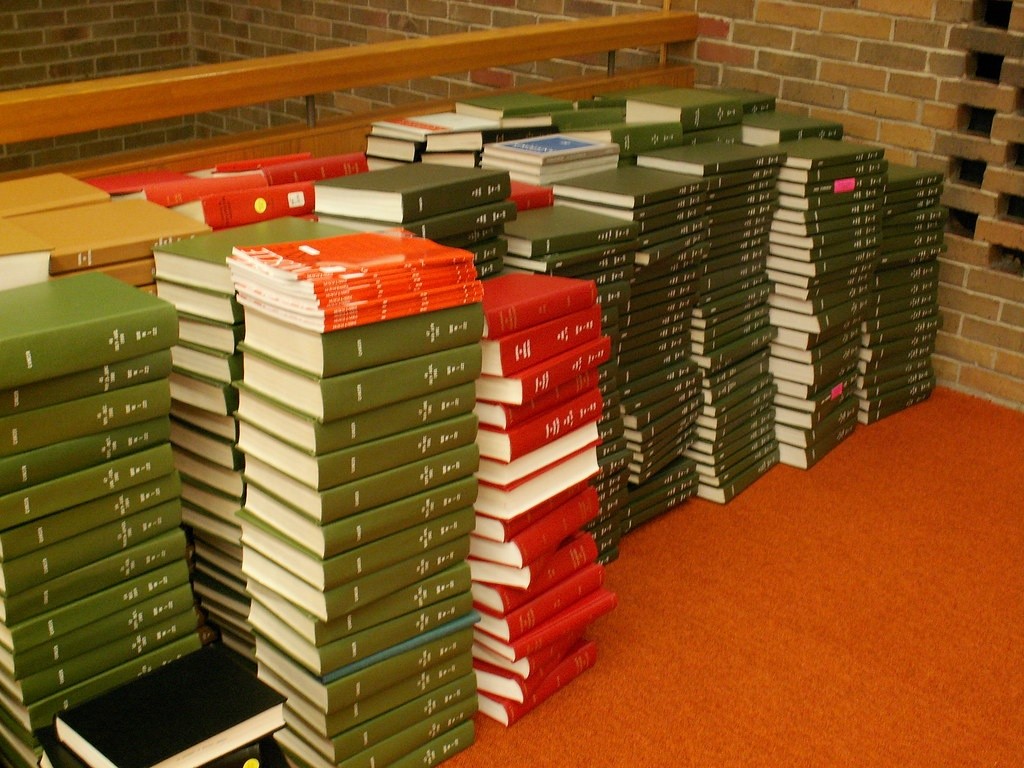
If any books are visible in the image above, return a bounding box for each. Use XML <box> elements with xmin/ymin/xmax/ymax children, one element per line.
<box><xmin>1</xmin><ymin>84</ymin><xmax>945</xmax><ymax>767</ymax></box>
